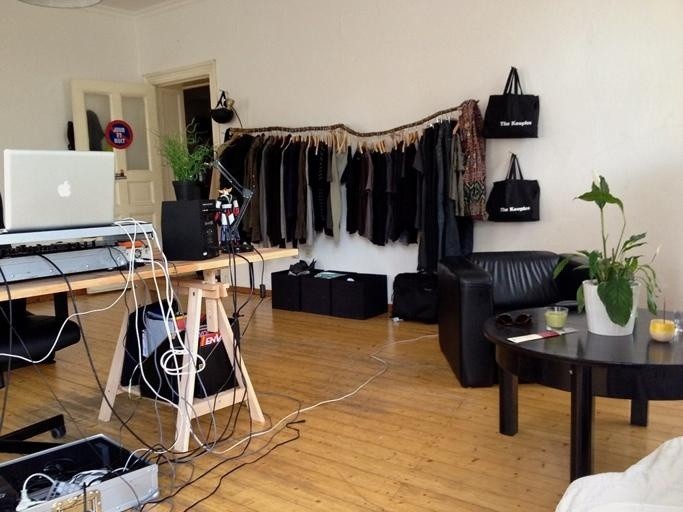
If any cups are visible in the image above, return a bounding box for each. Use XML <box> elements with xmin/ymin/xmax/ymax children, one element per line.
<box><xmin>650</xmin><ymin>318</ymin><xmax>674</xmax><ymax>341</ymax></box>
<box><xmin>543</xmin><ymin>306</ymin><xmax>568</xmax><ymax>330</ymax></box>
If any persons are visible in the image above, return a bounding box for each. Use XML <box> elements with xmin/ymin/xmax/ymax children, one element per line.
<box><xmin>215</xmin><ymin>182</ymin><xmax>241</xmax><ymax>247</ymax></box>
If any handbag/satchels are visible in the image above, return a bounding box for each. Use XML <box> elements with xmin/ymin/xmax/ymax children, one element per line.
<box><xmin>389</xmin><ymin>269</ymin><xmax>441</xmax><ymax>325</ymax></box>
<box><xmin>485</xmin><ymin>154</ymin><xmax>542</xmax><ymax>223</ymax></box>
<box><xmin>480</xmin><ymin>67</ymin><xmax>541</xmax><ymax>139</ymax></box>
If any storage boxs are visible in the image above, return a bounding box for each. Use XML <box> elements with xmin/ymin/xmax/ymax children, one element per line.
<box><xmin>269</xmin><ymin>267</ymin><xmax>388</xmax><ymax>324</ymax></box>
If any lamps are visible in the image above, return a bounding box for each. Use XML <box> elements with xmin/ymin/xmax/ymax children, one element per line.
<box><xmin>175</xmin><ymin>144</ymin><xmax>257</xmax><ymax>256</ymax></box>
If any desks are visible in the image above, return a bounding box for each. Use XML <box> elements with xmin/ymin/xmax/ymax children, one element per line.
<box><xmin>478</xmin><ymin>305</ymin><xmax>682</xmax><ymax>482</ymax></box>
<box><xmin>1</xmin><ymin>237</ymin><xmax>300</xmax><ymax>453</ymax></box>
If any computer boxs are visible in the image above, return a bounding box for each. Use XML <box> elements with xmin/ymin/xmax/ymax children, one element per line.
<box><xmin>161</xmin><ymin>198</ymin><xmax>217</xmax><ymax>262</ymax></box>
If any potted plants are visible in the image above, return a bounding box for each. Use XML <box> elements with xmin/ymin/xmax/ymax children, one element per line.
<box><xmin>156</xmin><ymin>120</ymin><xmax>217</xmax><ymax>202</ymax></box>
<box><xmin>553</xmin><ymin>174</ymin><xmax>662</xmax><ymax>338</ymax></box>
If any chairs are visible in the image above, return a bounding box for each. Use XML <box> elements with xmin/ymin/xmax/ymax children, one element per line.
<box><xmin>0</xmin><ymin>298</ymin><xmax>82</xmax><ymax>454</ymax></box>
<box><xmin>434</xmin><ymin>245</ymin><xmax>593</xmax><ymax>389</ymax></box>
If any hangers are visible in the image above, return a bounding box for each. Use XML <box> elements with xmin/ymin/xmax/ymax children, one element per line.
<box><xmin>227</xmin><ymin>107</ymin><xmax>471</xmax><ymax>154</ymax></box>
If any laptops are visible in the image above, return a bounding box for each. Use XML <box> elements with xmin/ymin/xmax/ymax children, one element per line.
<box><xmin>0</xmin><ymin>149</ymin><xmax>116</xmax><ymax>234</ymax></box>
